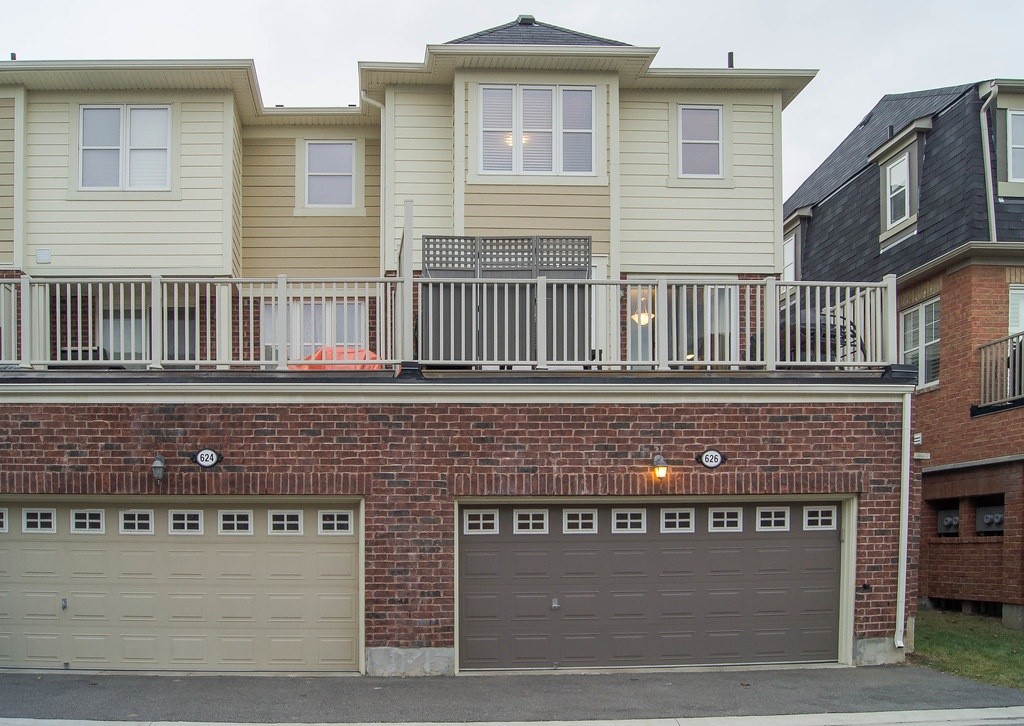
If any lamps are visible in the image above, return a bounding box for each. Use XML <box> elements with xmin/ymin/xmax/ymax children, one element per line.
<box><xmin>653</xmin><ymin>454</ymin><xmax>668</xmax><ymax>481</ymax></box>
<box><xmin>630</xmin><ymin>294</ymin><xmax>655</xmax><ymax>326</ymax></box>
<box><xmin>149</xmin><ymin>452</ymin><xmax>167</xmax><ymax>483</ymax></box>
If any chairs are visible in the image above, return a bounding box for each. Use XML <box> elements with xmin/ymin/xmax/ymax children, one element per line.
<box><xmin>49</xmin><ymin>347</ymin><xmax>125</xmax><ymax>370</ymax></box>
<box><xmin>290</xmin><ymin>343</ymin><xmax>381</xmax><ymax>372</ymax></box>
